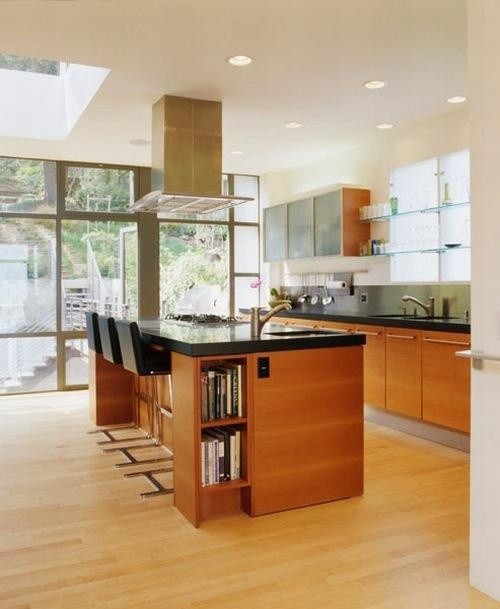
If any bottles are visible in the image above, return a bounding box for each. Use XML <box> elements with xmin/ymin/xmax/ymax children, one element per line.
<box><xmin>359</xmin><ymin>238</ymin><xmax>390</xmax><ymax>255</ymax></box>
<box><xmin>358</xmin><ymin>196</ymin><xmax>399</xmax><ymax>222</ymax></box>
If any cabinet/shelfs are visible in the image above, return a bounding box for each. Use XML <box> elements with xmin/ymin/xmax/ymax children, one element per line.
<box><xmin>354</xmin><ymin>202</ymin><xmax>471</xmax><ymax>257</ymax></box>
<box><xmin>197</xmin><ymin>357</ymin><xmax>254</xmax><ymax>532</ymax></box>
<box><xmin>266</xmin><ymin>316</ymin><xmax>471</xmax><ymax>435</ymax></box>
<box><xmin>264</xmin><ymin>186</ymin><xmax>370</xmax><ymax>262</ymax></box>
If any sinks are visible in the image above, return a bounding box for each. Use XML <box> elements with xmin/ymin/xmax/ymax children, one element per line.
<box><xmin>403</xmin><ymin>316</ymin><xmax>462</xmax><ymax>320</ymax></box>
<box><xmin>263</xmin><ymin>330</ymin><xmax>343</xmax><ymax>337</ymax></box>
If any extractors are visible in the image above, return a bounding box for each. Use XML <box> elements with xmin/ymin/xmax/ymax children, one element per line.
<box><xmin>125</xmin><ymin>94</ymin><xmax>255</xmax><ymax>217</ymax></box>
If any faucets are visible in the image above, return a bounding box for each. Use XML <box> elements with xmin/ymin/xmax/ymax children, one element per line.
<box><xmin>442</xmin><ymin>296</ymin><xmax>458</xmax><ymax>317</ymax></box>
<box><xmin>401</xmin><ymin>295</ymin><xmax>435</xmax><ymax>317</ymax></box>
<box><xmin>251</xmin><ymin>302</ymin><xmax>293</xmax><ymax>338</ymax></box>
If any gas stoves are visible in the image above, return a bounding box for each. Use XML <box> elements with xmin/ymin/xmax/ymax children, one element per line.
<box><xmin>163</xmin><ymin>312</ymin><xmax>252</xmax><ymax>325</ymax></box>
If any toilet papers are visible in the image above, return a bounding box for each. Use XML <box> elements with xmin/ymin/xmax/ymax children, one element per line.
<box><xmin>326</xmin><ymin>281</ymin><xmax>348</xmax><ymax>290</ymax></box>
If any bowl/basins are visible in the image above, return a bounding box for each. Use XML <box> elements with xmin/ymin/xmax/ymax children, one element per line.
<box><xmin>268</xmin><ymin>300</ymin><xmax>292</xmax><ymax>309</ymax></box>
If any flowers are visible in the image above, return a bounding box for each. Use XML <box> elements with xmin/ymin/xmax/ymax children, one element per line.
<box><xmin>250</xmin><ymin>278</ymin><xmax>279</xmax><ymax>295</ymax></box>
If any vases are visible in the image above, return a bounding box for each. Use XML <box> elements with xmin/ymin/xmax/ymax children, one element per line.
<box><xmin>272</xmin><ymin>295</ymin><xmax>281</xmax><ymax>301</ymax></box>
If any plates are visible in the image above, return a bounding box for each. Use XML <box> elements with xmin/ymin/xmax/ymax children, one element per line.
<box><xmin>445</xmin><ymin>243</ymin><xmax>462</xmax><ymax>248</ymax></box>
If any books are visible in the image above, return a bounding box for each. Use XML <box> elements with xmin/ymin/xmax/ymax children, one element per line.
<box><xmin>198</xmin><ymin>360</ymin><xmax>243</xmax><ymax>488</ymax></box>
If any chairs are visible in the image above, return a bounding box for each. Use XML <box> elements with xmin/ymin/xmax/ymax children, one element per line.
<box><xmin>86</xmin><ymin>312</ymin><xmax>171</xmax><ymax>498</ymax></box>
